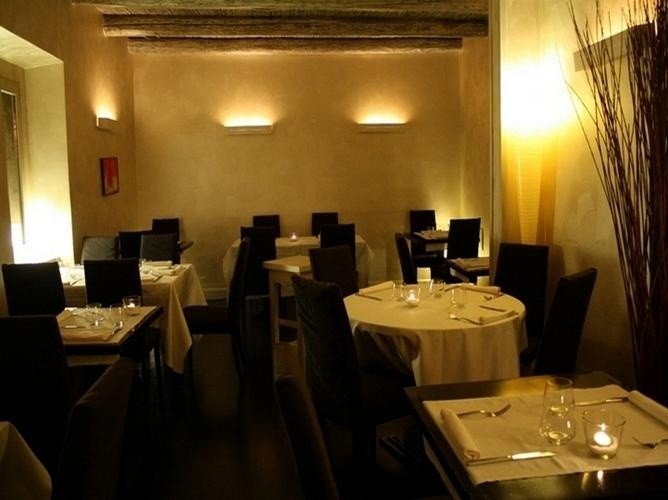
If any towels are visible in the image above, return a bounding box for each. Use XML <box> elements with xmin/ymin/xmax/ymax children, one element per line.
<box><xmin>461</xmin><ymin>283</ymin><xmax>503</xmax><ymax>296</ymax></box>
<box><xmin>626</xmin><ymin>388</ymin><xmax>668</xmax><ymax>432</ymax></box>
<box><xmin>440</xmin><ymin>407</ymin><xmax>481</xmax><ymax>463</ymax></box>
<box><xmin>479</xmin><ymin>310</ymin><xmax>516</xmax><ymax>324</ymax></box>
<box><xmin>62</xmin><ymin>332</ymin><xmax>107</xmax><ymax>342</ymax></box>
<box><xmin>357</xmin><ymin>279</ymin><xmax>395</xmax><ymax>294</ymax></box>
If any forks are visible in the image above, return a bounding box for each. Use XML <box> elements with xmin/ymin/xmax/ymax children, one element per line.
<box><xmin>455</xmin><ymin>403</ymin><xmax>512</xmax><ymax>417</ymax></box>
<box><xmin>632</xmin><ymin>436</ymin><xmax>668</xmax><ymax>450</ymax></box>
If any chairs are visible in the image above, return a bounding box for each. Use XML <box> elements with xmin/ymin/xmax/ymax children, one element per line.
<box><xmin>80</xmin><ymin>235</ymin><xmax>120</xmax><ymax>265</ymax></box>
<box><xmin>1</xmin><ymin>261</ymin><xmax>68</xmax><ymax>315</ymax></box>
<box><xmin>409</xmin><ymin>209</ymin><xmax>437</xmax><ymax>253</ymax></box>
<box><xmin>392</xmin><ymin>232</ymin><xmax>418</xmax><ymax>282</ymax></box>
<box><xmin>522</xmin><ymin>267</ymin><xmax>599</xmax><ymax>376</ymax></box>
<box><xmin>495</xmin><ymin>242</ymin><xmax>549</xmax><ymax>296</ymax></box>
<box><xmin>252</xmin><ymin>215</ymin><xmax>280</xmax><ymax>237</ymax></box>
<box><xmin>84</xmin><ymin>258</ymin><xmax>143</xmax><ymax>307</ymax></box>
<box><xmin>230</xmin><ymin>236</ymin><xmax>278</xmax><ymax>335</ymax></box>
<box><xmin>140</xmin><ymin>233</ymin><xmax>180</xmax><ymax>264</ymax></box>
<box><xmin>2</xmin><ymin>315</ymin><xmax>132</xmax><ymax>454</ymax></box>
<box><xmin>308</xmin><ymin>246</ymin><xmax>358</xmax><ymax>296</ymax></box>
<box><xmin>273</xmin><ymin>339</ymin><xmax>452</xmax><ymax>499</ymax></box>
<box><xmin>446</xmin><ymin>217</ymin><xmax>481</xmax><ymax>285</ymax></box>
<box><xmin>320</xmin><ymin>223</ymin><xmax>356</xmax><ymax>249</ymax></box>
<box><xmin>290</xmin><ymin>274</ymin><xmax>423</xmax><ymax>459</ymax></box>
<box><xmin>151</xmin><ymin>219</ymin><xmax>181</xmax><ymax>262</ymax></box>
<box><xmin>240</xmin><ymin>226</ymin><xmax>275</xmax><ymax>239</ymax></box>
<box><xmin>311</xmin><ymin>212</ymin><xmax>338</xmax><ymax>235</ymax></box>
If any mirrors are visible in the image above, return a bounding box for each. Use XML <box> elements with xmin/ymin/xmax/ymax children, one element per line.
<box><xmin>0</xmin><ymin>91</ymin><xmax>25</xmax><ymax>311</ymax></box>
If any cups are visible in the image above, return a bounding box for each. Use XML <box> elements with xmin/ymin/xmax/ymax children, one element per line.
<box><xmin>581</xmin><ymin>407</ymin><xmax>627</xmax><ymax>461</ymax></box>
<box><xmin>543</xmin><ymin>377</ymin><xmax>575</xmax><ymax>414</ymax></box>
<box><xmin>109</xmin><ymin>304</ymin><xmax>123</xmax><ymax>323</ymax></box>
<box><xmin>449</xmin><ymin>286</ymin><xmax>466</xmax><ymax>307</ymax></box>
<box><xmin>122</xmin><ymin>295</ymin><xmax>141</xmax><ymax>316</ymax></box>
<box><xmin>403</xmin><ymin>285</ymin><xmax>421</xmax><ymax>307</ymax></box>
<box><xmin>434</xmin><ymin>279</ymin><xmax>444</xmax><ymax>291</ymax></box>
<box><xmin>85</xmin><ymin>303</ymin><xmax>104</xmax><ymax>330</ymax></box>
<box><xmin>537</xmin><ymin>405</ymin><xmax>577</xmax><ymax>447</ymax></box>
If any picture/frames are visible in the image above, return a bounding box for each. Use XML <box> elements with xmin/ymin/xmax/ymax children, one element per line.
<box><xmin>101</xmin><ymin>156</ymin><xmax>120</xmax><ymax>196</ymax></box>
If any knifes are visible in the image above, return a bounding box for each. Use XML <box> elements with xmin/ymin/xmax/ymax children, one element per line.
<box><xmin>355</xmin><ymin>293</ymin><xmax>382</xmax><ymax>301</ymax></box>
<box><xmin>479</xmin><ymin>305</ymin><xmax>519</xmax><ymax>315</ymax></box>
<box><xmin>464</xmin><ymin>449</ymin><xmax>559</xmax><ymax>466</ymax></box>
<box><xmin>575</xmin><ymin>397</ymin><xmax>628</xmax><ymax>406</ymax></box>
